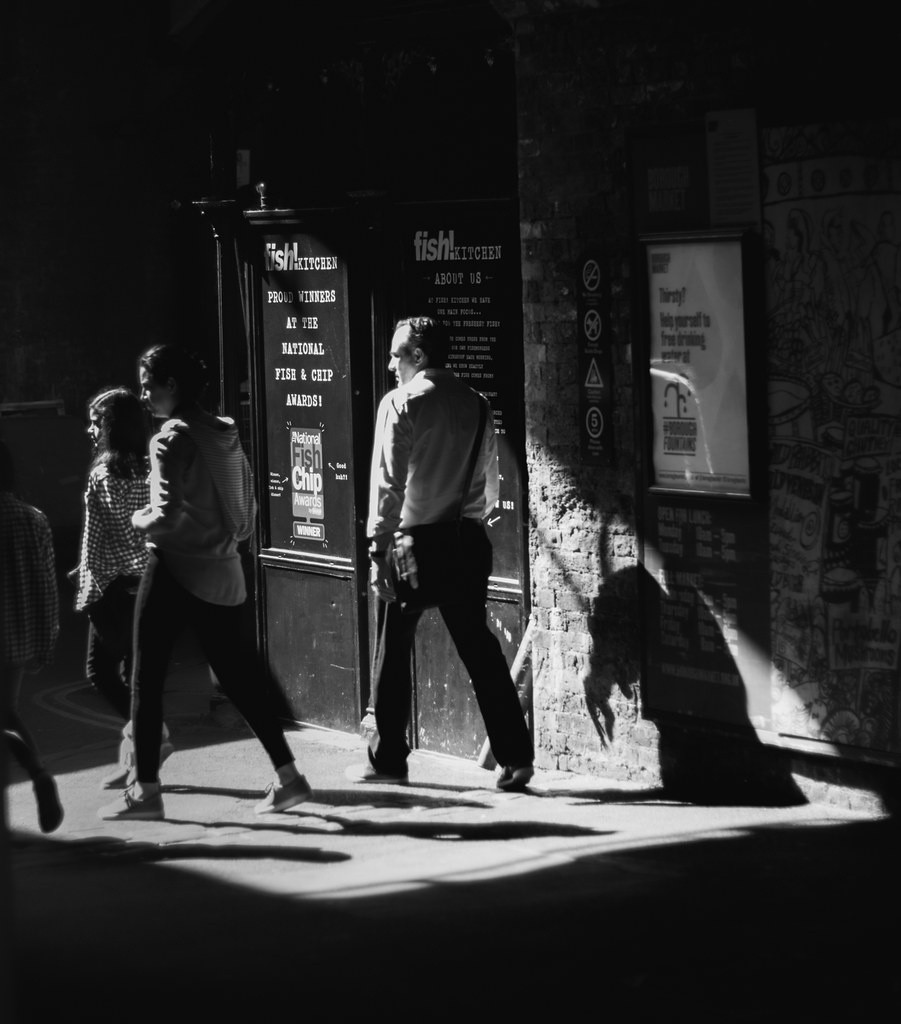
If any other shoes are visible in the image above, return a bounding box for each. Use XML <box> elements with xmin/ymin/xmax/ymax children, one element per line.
<box><xmin>104</xmin><ymin>768</ymin><xmax>129</xmax><ymax>787</ymax></box>
<box><xmin>33</xmin><ymin>775</ymin><xmax>62</xmax><ymax>834</ymax></box>
<box><xmin>159</xmin><ymin>734</ymin><xmax>173</xmax><ymax>765</ymax></box>
<box><xmin>367</xmin><ymin>730</ymin><xmax>407</xmax><ymax>778</ymax></box>
<box><xmin>496</xmin><ymin>765</ymin><xmax>535</xmax><ymax>787</ymax></box>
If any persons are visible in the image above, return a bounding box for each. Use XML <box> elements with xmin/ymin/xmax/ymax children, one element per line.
<box><xmin>0</xmin><ymin>441</ymin><xmax>64</xmax><ymax>832</ymax></box>
<box><xmin>342</xmin><ymin>316</ymin><xmax>535</xmax><ymax>787</ymax></box>
<box><xmin>98</xmin><ymin>345</ymin><xmax>313</xmax><ymax>821</ymax></box>
<box><xmin>74</xmin><ymin>387</ymin><xmax>176</xmax><ymax>789</ymax></box>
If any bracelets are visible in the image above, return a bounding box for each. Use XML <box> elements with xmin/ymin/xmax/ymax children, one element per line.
<box><xmin>369</xmin><ymin>551</ymin><xmax>386</xmax><ymax>557</ymax></box>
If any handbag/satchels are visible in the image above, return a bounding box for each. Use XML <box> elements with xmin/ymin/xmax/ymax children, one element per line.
<box><xmin>391</xmin><ymin>519</ymin><xmax>476</xmax><ymax>614</ymax></box>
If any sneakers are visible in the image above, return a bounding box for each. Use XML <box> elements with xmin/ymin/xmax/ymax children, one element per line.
<box><xmin>256</xmin><ymin>774</ymin><xmax>314</xmax><ymax>814</ymax></box>
<box><xmin>97</xmin><ymin>785</ymin><xmax>165</xmax><ymax>821</ymax></box>
